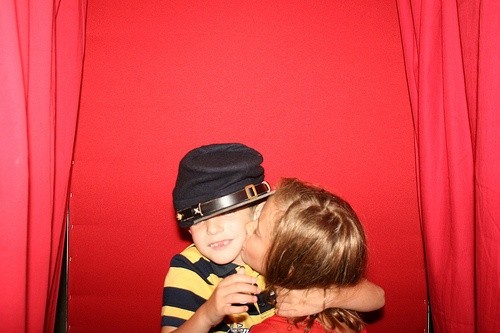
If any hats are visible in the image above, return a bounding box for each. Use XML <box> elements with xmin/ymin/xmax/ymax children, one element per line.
<box><xmin>172</xmin><ymin>143</ymin><xmax>279</xmax><ymax>229</ymax></box>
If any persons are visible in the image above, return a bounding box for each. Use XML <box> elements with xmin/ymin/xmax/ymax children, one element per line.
<box><xmin>241</xmin><ymin>176</ymin><xmax>368</xmax><ymax>333</ymax></box>
<box><xmin>161</xmin><ymin>143</ymin><xmax>385</xmax><ymax>333</ymax></box>
